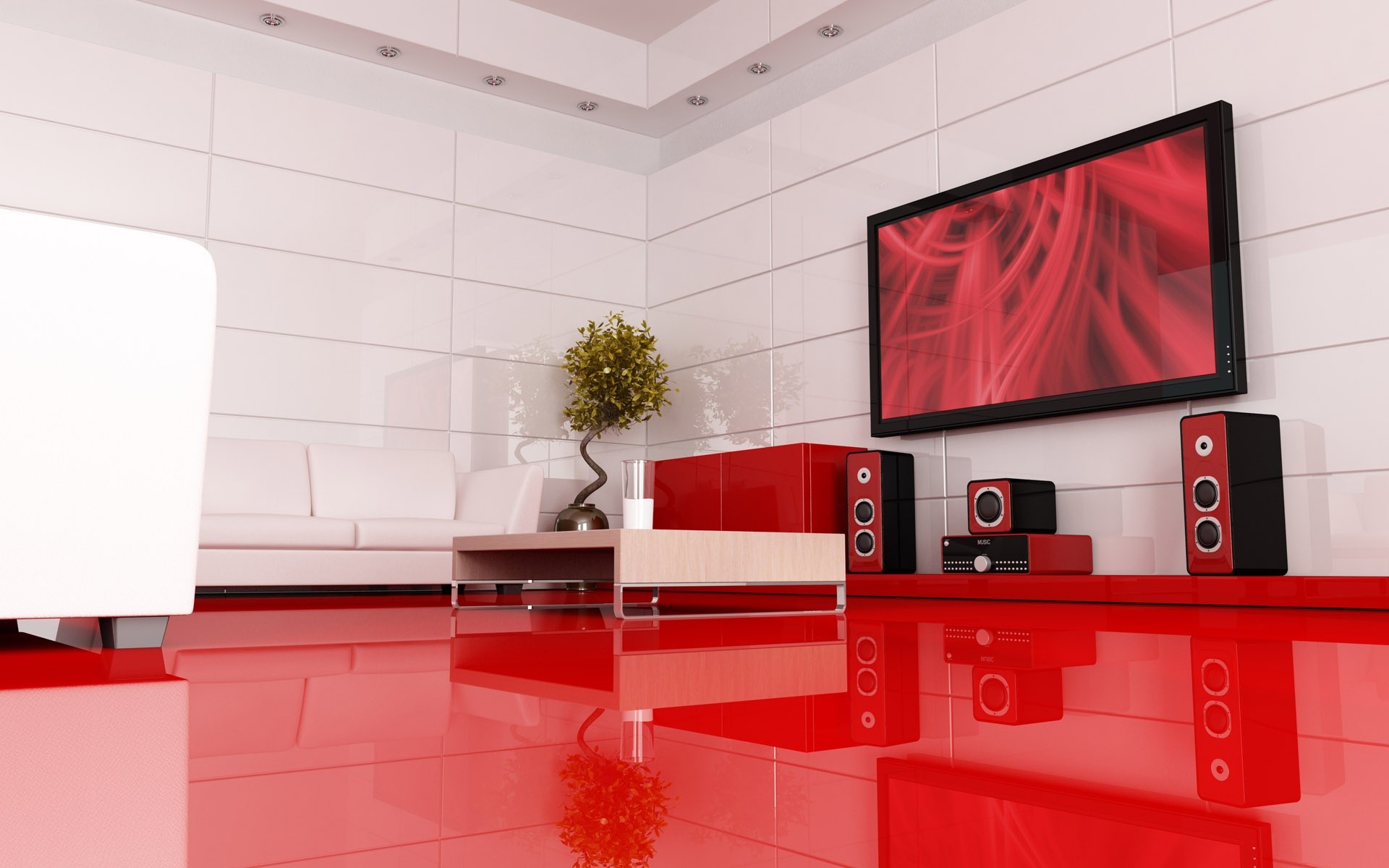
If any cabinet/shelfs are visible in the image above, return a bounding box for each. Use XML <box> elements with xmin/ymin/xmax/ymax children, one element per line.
<box><xmin>654</xmin><ymin>443</ymin><xmax>869</xmax><ymax>532</ymax></box>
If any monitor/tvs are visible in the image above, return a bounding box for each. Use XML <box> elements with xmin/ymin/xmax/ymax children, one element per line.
<box><xmin>867</xmin><ymin>100</ymin><xmax>1247</xmax><ymax>438</ymax></box>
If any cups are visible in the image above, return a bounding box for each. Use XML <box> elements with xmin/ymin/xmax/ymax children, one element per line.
<box><xmin>622</xmin><ymin>460</ymin><xmax>654</xmax><ymax>529</ymax></box>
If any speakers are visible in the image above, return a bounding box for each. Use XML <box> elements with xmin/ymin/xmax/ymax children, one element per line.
<box><xmin>971</xmin><ymin>664</ymin><xmax>1063</xmax><ymax>727</ymax></box>
<box><xmin>1180</xmin><ymin>409</ymin><xmax>1288</xmax><ymax>575</ymax></box>
<box><xmin>967</xmin><ymin>478</ymin><xmax>1057</xmax><ymax>535</ymax></box>
<box><xmin>1188</xmin><ymin>634</ymin><xmax>1300</xmax><ymax>808</ymax></box>
<box><xmin>845</xmin><ymin>449</ymin><xmax>917</xmax><ymax>575</ymax></box>
<box><xmin>847</xmin><ymin>615</ymin><xmax>921</xmax><ymax>746</ymax></box>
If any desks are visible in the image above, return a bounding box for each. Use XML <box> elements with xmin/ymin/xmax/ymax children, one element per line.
<box><xmin>451</xmin><ymin>529</ymin><xmax>847</xmax><ymax>620</ymax></box>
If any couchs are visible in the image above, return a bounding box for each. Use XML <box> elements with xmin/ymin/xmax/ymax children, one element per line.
<box><xmin>0</xmin><ymin>208</ymin><xmax>219</xmax><ymax>650</ymax></box>
<box><xmin>195</xmin><ymin>436</ymin><xmax>544</xmax><ymax>594</ymax></box>
